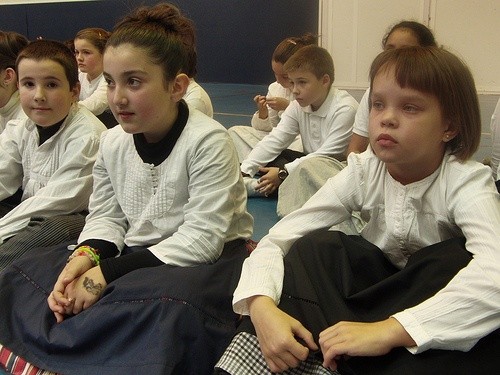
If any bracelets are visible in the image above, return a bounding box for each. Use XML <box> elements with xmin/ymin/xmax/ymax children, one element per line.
<box><xmin>67</xmin><ymin>246</ymin><xmax>99</xmax><ymax>266</ymax></box>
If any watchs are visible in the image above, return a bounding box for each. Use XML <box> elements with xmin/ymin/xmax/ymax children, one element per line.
<box><xmin>278</xmin><ymin>164</ymin><xmax>288</xmax><ymax>181</ymax></box>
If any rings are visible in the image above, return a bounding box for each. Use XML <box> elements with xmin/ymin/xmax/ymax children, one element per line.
<box><xmin>69</xmin><ymin>299</ymin><xmax>73</xmax><ymax>302</ymax></box>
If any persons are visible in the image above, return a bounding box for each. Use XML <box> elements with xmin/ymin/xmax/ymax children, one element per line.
<box><xmin>0</xmin><ymin>3</ymin><xmax>255</xmax><ymax>374</ymax></box>
<box><xmin>0</xmin><ymin>31</ymin><xmax>43</xmax><ymax>134</ymax></box>
<box><xmin>74</xmin><ymin>27</ymin><xmax>119</xmax><ymax>130</ymax></box>
<box><xmin>276</xmin><ymin>21</ymin><xmax>439</xmax><ymax>219</ymax></box>
<box><xmin>181</xmin><ymin>47</ymin><xmax>213</xmax><ymax>118</ymax></box>
<box><xmin>228</xmin><ymin>32</ymin><xmax>318</xmax><ymax>164</ymax></box>
<box><xmin>0</xmin><ymin>40</ymin><xmax>108</xmax><ymax>273</ymax></box>
<box><xmin>213</xmin><ymin>46</ymin><xmax>500</xmax><ymax>375</ymax></box>
<box><xmin>240</xmin><ymin>45</ymin><xmax>360</xmax><ymax>198</ymax></box>
